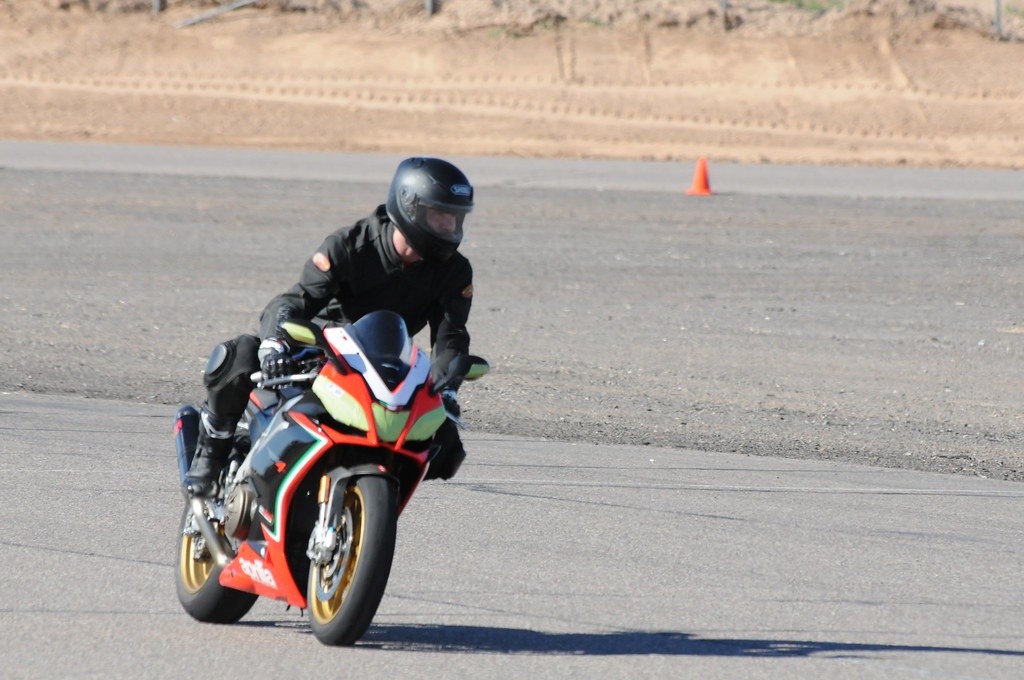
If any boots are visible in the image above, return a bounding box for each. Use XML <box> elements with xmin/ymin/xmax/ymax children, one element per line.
<box><xmin>181</xmin><ymin>401</ymin><xmax>242</xmax><ymax>498</ymax></box>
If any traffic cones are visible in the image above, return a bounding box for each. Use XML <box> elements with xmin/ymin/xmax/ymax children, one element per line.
<box><xmin>685</xmin><ymin>156</ymin><xmax>714</xmax><ymax>194</ymax></box>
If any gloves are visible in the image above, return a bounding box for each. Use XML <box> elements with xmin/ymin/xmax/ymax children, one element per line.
<box><xmin>257</xmin><ymin>336</ymin><xmax>294</xmax><ymax>391</ymax></box>
<box><xmin>441</xmin><ymin>390</ymin><xmax>461</xmax><ymax>418</ymax></box>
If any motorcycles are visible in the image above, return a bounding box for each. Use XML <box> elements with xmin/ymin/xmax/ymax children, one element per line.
<box><xmin>171</xmin><ymin>318</ymin><xmax>490</xmax><ymax>646</ymax></box>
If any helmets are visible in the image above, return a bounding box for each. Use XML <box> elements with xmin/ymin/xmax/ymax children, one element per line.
<box><xmin>386</xmin><ymin>157</ymin><xmax>474</xmax><ymax>264</ymax></box>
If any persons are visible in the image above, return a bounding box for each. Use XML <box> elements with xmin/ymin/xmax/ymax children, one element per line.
<box><xmin>183</xmin><ymin>157</ymin><xmax>475</xmax><ymax>494</ymax></box>
